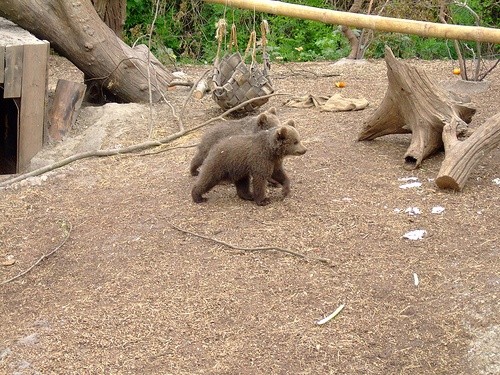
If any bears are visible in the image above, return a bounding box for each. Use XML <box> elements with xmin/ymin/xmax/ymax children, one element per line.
<box><xmin>190</xmin><ymin>119</ymin><xmax>308</xmax><ymax>207</ymax></box>
<box><xmin>190</xmin><ymin>106</ymin><xmax>281</xmax><ymax>177</ymax></box>
